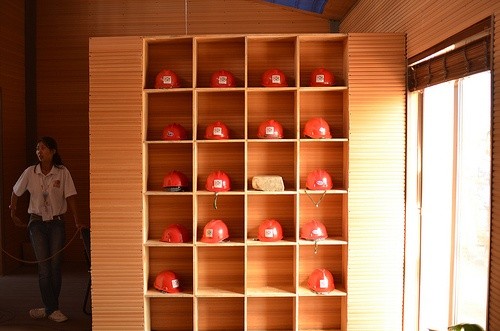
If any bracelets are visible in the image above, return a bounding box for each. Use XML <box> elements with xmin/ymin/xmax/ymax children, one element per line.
<box><xmin>75</xmin><ymin>224</ymin><xmax>82</xmax><ymax>228</ymax></box>
<box><xmin>9</xmin><ymin>205</ymin><xmax>17</xmax><ymax>210</ymax></box>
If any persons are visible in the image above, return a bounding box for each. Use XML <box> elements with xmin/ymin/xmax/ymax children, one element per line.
<box><xmin>11</xmin><ymin>138</ymin><xmax>85</xmax><ymax>321</ymax></box>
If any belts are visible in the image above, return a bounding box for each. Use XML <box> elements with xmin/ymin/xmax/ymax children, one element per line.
<box><xmin>27</xmin><ymin>213</ymin><xmax>64</xmax><ymax>232</ymax></box>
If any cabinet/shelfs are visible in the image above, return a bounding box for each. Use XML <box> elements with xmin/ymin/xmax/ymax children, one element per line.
<box><xmin>142</xmin><ymin>33</ymin><xmax>348</xmax><ymax>331</ymax></box>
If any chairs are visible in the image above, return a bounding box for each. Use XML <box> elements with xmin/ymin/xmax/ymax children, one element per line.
<box><xmin>80</xmin><ymin>227</ymin><xmax>92</xmax><ymax>315</ymax></box>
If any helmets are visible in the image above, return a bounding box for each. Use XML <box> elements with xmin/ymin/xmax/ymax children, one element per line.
<box><xmin>158</xmin><ymin>223</ymin><xmax>191</xmax><ymax>242</ymax></box>
<box><xmin>259</xmin><ymin>68</ymin><xmax>288</xmax><ymax>86</ymax></box>
<box><xmin>154</xmin><ymin>270</ymin><xmax>187</xmax><ymax>292</ymax></box>
<box><xmin>306</xmin><ymin>268</ymin><xmax>335</xmax><ymax>291</ymax></box>
<box><xmin>161</xmin><ymin>123</ymin><xmax>188</xmax><ymax>140</ymax></box>
<box><xmin>300</xmin><ymin>220</ymin><xmax>328</xmax><ymax>240</ymax></box>
<box><xmin>258</xmin><ymin>219</ymin><xmax>284</xmax><ymax>242</ymax></box>
<box><xmin>162</xmin><ymin>170</ymin><xmax>189</xmax><ymax>187</ymax></box>
<box><xmin>153</xmin><ymin>69</ymin><xmax>183</xmax><ymax>88</ymax></box>
<box><xmin>309</xmin><ymin>68</ymin><xmax>333</xmax><ymax>87</ymax></box>
<box><xmin>256</xmin><ymin>120</ymin><xmax>284</xmax><ymax>138</ymax></box>
<box><xmin>303</xmin><ymin>117</ymin><xmax>332</xmax><ymax>139</ymax></box>
<box><xmin>204</xmin><ymin>170</ymin><xmax>231</xmax><ymax>192</ymax></box>
<box><xmin>305</xmin><ymin>169</ymin><xmax>333</xmax><ymax>190</ymax></box>
<box><xmin>200</xmin><ymin>219</ymin><xmax>230</xmax><ymax>243</ymax></box>
<box><xmin>203</xmin><ymin>121</ymin><xmax>233</xmax><ymax>139</ymax></box>
<box><xmin>208</xmin><ymin>70</ymin><xmax>236</xmax><ymax>87</ymax></box>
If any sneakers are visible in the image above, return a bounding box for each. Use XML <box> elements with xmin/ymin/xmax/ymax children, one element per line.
<box><xmin>29</xmin><ymin>308</ymin><xmax>46</xmax><ymax>320</ymax></box>
<box><xmin>48</xmin><ymin>310</ymin><xmax>69</xmax><ymax>323</ymax></box>
